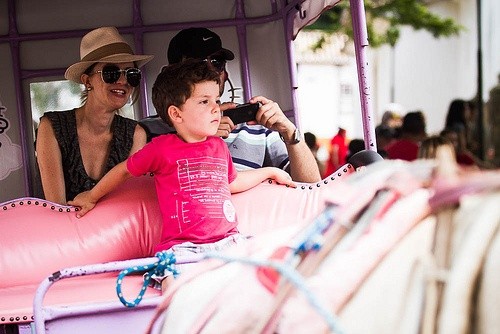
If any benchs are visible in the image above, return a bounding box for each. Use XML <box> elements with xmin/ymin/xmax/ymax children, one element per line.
<box><xmin>0</xmin><ymin>149</ymin><xmax>383</xmax><ymax>323</ymax></box>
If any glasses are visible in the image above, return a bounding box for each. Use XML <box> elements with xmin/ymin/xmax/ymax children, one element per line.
<box><xmin>88</xmin><ymin>63</ymin><xmax>143</xmax><ymax>87</ymax></box>
<box><xmin>181</xmin><ymin>54</ymin><xmax>227</xmax><ymax>71</ymax></box>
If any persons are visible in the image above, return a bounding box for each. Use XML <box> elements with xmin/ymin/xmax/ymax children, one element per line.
<box><xmin>323</xmin><ymin>128</ymin><xmax>347</xmax><ymax>180</ymax></box>
<box><xmin>66</xmin><ymin>59</ymin><xmax>297</xmax><ymax>277</ymax></box>
<box><xmin>304</xmin><ymin>132</ymin><xmax>324</xmax><ymax>176</ymax></box>
<box><xmin>346</xmin><ymin>74</ymin><xmax>500</xmax><ymax>170</ymax></box>
<box><xmin>137</xmin><ymin>27</ymin><xmax>322</xmax><ymax>183</ymax></box>
<box><xmin>36</xmin><ymin>26</ymin><xmax>147</xmax><ymax>206</ymax></box>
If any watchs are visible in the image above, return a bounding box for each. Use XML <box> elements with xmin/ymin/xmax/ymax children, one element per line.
<box><xmin>280</xmin><ymin>129</ymin><xmax>302</xmax><ymax>145</ymax></box>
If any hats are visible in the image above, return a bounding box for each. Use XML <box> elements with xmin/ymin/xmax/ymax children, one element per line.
<box><xmin>64</xmin><ymin>26</ymin><xmax>154</xmax><ymax>84</ymax></box>
<box><xmin>167</xmin><ymin>27</ymin><xmax>234</xmax><ymax>64</ymax></box>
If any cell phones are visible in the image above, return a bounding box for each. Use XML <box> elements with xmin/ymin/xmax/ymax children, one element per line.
<box><xmin>223</xmin><ymin>103</ymin><xmax>259</xmax><ymax>125</ymax></box>
